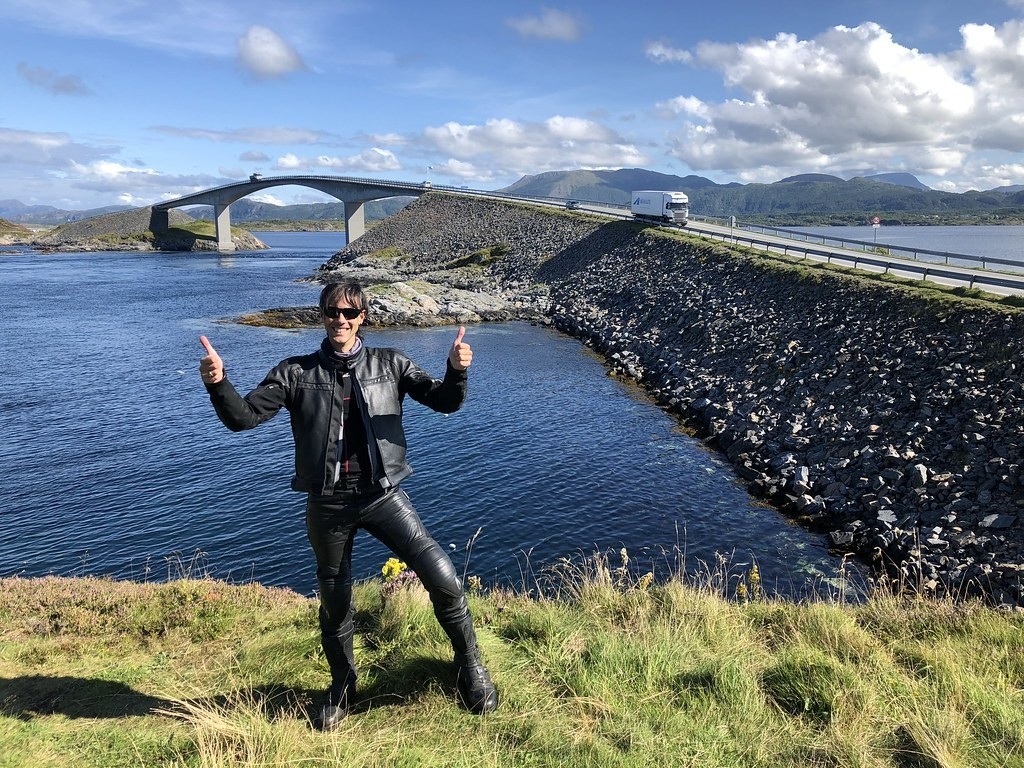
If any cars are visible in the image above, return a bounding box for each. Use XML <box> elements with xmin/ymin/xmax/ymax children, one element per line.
<box><xmin>566</xmin><ymin>200</ymin><xmax>582</xmax><ymax>210</ymax></box>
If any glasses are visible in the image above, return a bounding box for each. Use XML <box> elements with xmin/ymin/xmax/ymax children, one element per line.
<box><xmin>324</xmin><ymin>307</ymin><xmax>362</xmax><ymax>320</ymax></box>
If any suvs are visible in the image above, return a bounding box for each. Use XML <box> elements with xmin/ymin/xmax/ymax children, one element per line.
<box><xmin>420</xmin><ymin>181</ymin><xmax>432</xmax><ymax>188</ymax></box>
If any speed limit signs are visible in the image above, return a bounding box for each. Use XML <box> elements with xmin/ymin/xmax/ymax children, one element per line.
<box><xmin>873</xmin><ymin>216</ymin><xmax>880</xmax><ymax>224</ymax></box>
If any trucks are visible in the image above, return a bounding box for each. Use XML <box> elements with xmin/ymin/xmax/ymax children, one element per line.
<box><xmin>250</xmin><ymin>173</ymin><xmax>262</xmax><ymax>182</ymax></box>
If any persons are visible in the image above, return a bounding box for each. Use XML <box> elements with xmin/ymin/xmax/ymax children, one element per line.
<box><xmin>197</xmin><ymin>281</ymin><xmax>507</xmax><ymax>731</ymax></box>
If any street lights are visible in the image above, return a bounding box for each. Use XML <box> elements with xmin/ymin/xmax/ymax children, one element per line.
<box><xmin>425</xmin><ymin>165</ymin><xmax>433</xmax><ymax>192</ymax></box>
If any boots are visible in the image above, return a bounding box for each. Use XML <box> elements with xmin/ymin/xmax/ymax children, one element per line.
<box><xmin>319</xmin><ymin>621</ymin><xmax>356</xmax><ymax>725</ymax></box>
<box><xmin>438</xmin><ymin>609</ymin><xmax>497</xmax><ymax>713</ymax></box>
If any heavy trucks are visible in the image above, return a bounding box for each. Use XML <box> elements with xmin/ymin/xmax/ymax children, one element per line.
<box><xmin>631</xmin><ymin>190</ymin><xmax>689</xmax><ymax>226</ymax></box>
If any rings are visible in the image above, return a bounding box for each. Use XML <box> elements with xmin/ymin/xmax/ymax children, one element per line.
<box><xmin>208</xmin><ymin>371</ymin><xmax>213</xmax><ymax>378</ymax></box>
